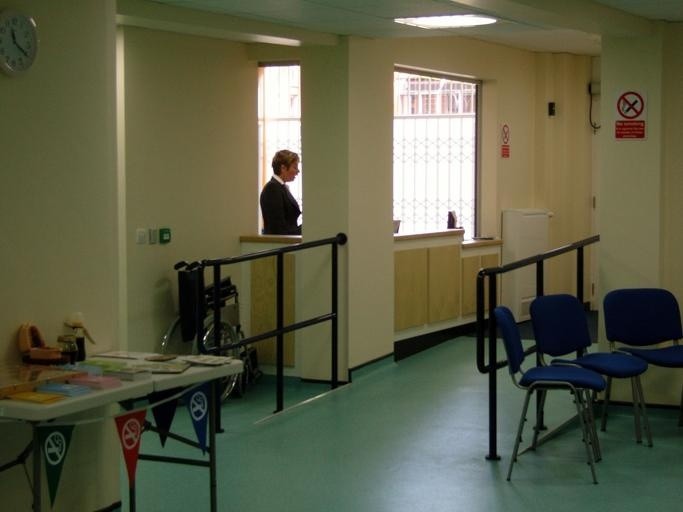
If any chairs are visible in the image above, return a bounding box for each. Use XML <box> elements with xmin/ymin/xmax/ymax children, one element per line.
<box><xmin>492</xmin><ymin>287</ymin><xmax>681</xmax><ymax>487</ymax></box>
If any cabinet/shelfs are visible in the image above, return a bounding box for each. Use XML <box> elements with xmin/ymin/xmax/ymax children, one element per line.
<box><xmin>461</xmin><ymin>251</ymin><xmax>499</xmax><ymax>319</ymax></box>
<box><xmin>392</xmin><ymin>243</ymin><xmax>462</xmax><ymax>330</ymax></box>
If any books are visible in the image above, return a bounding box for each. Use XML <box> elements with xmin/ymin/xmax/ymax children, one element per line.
<box><xmin>0</xmin><ymin>349</ymin><xmax>233</xmax><ymax>405</ymax></box>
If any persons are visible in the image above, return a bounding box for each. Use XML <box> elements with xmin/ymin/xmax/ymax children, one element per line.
<box><xmin>258</xmin><ymin>150</ymin><xmax>301</xmax><ymax>236</ymax></box>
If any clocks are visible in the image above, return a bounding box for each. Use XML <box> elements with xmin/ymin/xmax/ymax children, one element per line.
<box><xmin>0</xmin><ymin>10</ymin><xmax>40</xmax><ymax>80</ymax></box>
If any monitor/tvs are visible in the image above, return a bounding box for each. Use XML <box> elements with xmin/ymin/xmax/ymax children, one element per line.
<box><xmin>448</xmin><ymin>210</ymin><xmax>457</xmax><ymax>228</ymax></box>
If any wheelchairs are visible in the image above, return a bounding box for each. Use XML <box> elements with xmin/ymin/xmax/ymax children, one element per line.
<box><xmin>157</xmin><ymin>258</ymin><xmax>263</xmax><ymax>403</ymax></box>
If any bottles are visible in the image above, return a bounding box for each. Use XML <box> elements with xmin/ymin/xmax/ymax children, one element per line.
<box><xmin>57</xmin><ymin>319</ymin><xmax>88</xmax><ymax>367</ymax></box>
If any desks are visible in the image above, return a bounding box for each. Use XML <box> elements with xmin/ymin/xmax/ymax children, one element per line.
<box><xmin>1</xmin><ymin>347</ymin><xmax>246</xmax><ymax>512</ymax></box>
